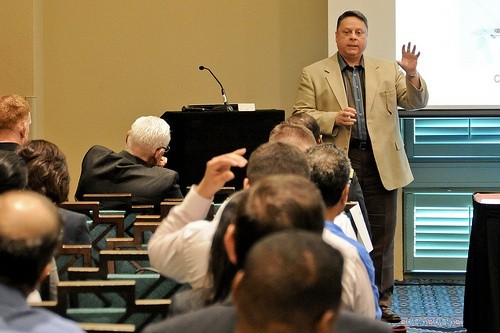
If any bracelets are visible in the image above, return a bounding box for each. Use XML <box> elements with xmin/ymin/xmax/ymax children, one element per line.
<box><xmin>410</xmin><ymin>74</ymin><xmax>417</xmax><ymax>78</ymax></box>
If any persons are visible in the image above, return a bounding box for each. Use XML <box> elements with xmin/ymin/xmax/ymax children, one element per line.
<box><xmin>74</xmin><ymin>116</ymin><xmax>183</xmax><ymax>215</ymax></box>
<box><xmin>293</xmin><ymin>11</ymin><xmax>427</xmax><ymax>324</ymax></box>
<box><xmin>141</xmin><ymin>114</ymin><xmax>392</xmax><ymax>333</ymax></box>
<box><xmin>0</xmin><ymin>94</ymin><xmax>94</xmax><ymax>333</ymax></box>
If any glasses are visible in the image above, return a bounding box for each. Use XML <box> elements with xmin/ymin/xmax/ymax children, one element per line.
<box><xmin>349</xmin><ymin>172</ymin><xmax>358</xmax><ymax>186</ymax></box>
<box><xmin>155</xmin><ymin>146</ymin><xmax>170</xmax><ymax>154</ymax></box>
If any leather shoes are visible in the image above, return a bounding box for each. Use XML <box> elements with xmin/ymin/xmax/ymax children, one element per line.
<box><xmin>381</xmin><ymin>306</ymin><xmax>401</xmax><ymax>321</ymax></box>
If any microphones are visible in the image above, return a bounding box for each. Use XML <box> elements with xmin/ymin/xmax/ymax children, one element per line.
<box><xmin>199</xmin><ymin>66</ymin><xmax>234</xmax><ymax>112</ymax></box>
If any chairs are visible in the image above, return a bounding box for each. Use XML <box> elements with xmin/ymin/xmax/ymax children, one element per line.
<box><xmin>29</xmin><ymin>187</ymin><xmax>237</xmax><ymax>333</ymax></box>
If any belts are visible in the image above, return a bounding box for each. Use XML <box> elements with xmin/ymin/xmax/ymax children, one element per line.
<box><xmin>348</xmin><ymin>138</ymin><xmax>372</xmax><ymax>151</ymax></box>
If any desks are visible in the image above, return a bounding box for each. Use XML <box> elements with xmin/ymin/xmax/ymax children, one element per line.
<box><xmin>158</xmin><ymin>110</ymin><xmax>285</xmax><ymax>195</ymax></box>
<box><xmin>463</xmin><ymin>192</ymin><xmax>500</xmax><ymax>333</ymax></box>
